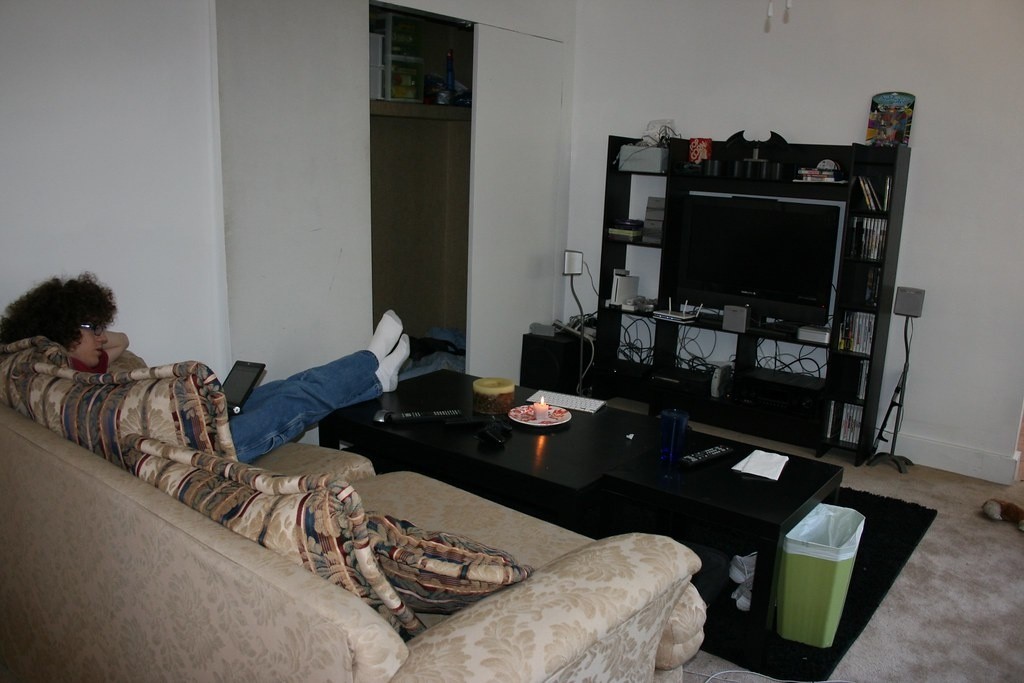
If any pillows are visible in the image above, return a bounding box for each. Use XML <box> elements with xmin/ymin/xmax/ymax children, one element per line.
<box><xmin>1</xmin><ymin>336</ymin><xmax>536</xmax><ymax>642</ymax></box>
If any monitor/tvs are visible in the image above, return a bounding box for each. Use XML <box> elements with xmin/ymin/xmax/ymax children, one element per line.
<box><xmin>679</xmin><ymin>194</ymin><xmax>840</xmax><ymax>337</ymax></box>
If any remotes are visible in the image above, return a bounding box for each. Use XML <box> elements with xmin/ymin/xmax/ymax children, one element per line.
<box><xmin>678</xmin><ymin>445</ymin><xmax>734</xmax><ymax>470</ymax></box>
<box><xmin>384</xmin><ymin>409</ymin><xmax>462</xmax><ymax>427</ymax></box>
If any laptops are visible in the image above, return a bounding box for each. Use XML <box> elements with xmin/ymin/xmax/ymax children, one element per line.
<box><xmin>220</xmin><ymin>361</ymin><xmax>267</xmax><ymax>420</ymax></box>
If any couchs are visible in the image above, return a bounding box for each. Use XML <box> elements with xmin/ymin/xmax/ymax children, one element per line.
<box><xmin>0</xmin><ymin>333</ymin><xmax>705</xmax><ymax>683</ymax></box>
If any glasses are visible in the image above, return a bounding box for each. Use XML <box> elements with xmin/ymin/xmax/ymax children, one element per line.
<box><xmin>78</xmin><ymin>323</ymin><xmax>108</xmax><ymax>335</ymax></box>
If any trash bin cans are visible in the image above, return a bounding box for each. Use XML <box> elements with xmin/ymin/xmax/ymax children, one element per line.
<box><xmin>777</xmin><ymin>502</ymin><xmax>866</xmax><ymax>649</ymax></box>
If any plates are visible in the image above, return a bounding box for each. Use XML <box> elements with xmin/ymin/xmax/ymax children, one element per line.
<box><xmin>508</xmin><ymin>405</ymin><xmax>572</xmax><ymax>427</ymax></box>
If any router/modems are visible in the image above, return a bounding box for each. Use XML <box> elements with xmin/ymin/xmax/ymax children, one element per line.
<box><xmin>653</xmin><ymin>297</ymin><xmax>703</xmax><ymax>324</ymax></box>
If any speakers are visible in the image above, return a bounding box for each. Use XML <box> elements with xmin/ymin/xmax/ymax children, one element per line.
<box><xmin>893</xmin><ymin>286</ymin><xmax>925</xmax><ymax>318</ymax></box>
<box><xmin>722</xmin><ymin>304</ymin><xmax>751</xmax><ymax>334</ymax></box>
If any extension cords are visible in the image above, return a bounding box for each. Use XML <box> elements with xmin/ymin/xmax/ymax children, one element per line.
<box><xmin>553</xmin><ymin>320</ymin><xmax>593</xmax><ymax>342</ymax></box>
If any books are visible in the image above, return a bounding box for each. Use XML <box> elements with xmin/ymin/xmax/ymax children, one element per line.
<box><xmin>835</xmin><ymin>310</ymin><xmax>875</xmax><ymax>355</ymax></box>
<box><xmin>844</xmin><ymin>215</ymin><xmax>887</xmax><ymax>261</ymax></box>
<box><xmin>791</xmin><ymin>168</ymin><xmax>848</xmax><ymax>183</ymax></box>
<box><xmin>863</xmin><ymin>265</ymin><xmax>881</xmax><ymax>309</ymax></box>
<box><xmin>857</xmin><ymin>360</ymin><xmax>868</xmax><ymax>400</ymax></box>
<box><xmin>857</xmin><ymin>175</ymin><xmax>893</xmax><ymax>213</ymax></box>
<box><xmin>825</xmin><ymin>400</ymin><xmax>863</xmax><ymax>444</ymax></box>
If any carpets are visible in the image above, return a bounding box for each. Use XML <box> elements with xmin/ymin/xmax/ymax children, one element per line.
<box><xmin>339</xmin><ymin>441</ymin><xmax>937</xmax><ymax>683</ymax></box>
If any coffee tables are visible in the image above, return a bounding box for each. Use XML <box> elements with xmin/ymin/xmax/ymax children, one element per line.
<box><xmin>604</xmin><ymin>428</ymin><xmax>844</xmax><ymax>635</ymax></box>
<box><xmin>319</xmin><ymin>369</ymin><xmax>693</xmax><ymax>531</ymax></box>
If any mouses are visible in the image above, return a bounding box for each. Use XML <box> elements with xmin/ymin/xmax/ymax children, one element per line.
<box><xmin>373</xmin><ymin>410</ymin><xmax>390</xmax><ymax>423</ymax></box>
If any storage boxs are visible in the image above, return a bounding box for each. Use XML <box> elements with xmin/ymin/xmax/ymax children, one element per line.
<box><xmin>643</xmin><ymin>197</ymin><xmax>664</xmax><ymax>244</ymax></box>
<box><xmin>619</xmin><ymin>146</ymin><xmax>669</xmax><ymax>174</ymax></box>
<box><xmin>370</xmin><ymin>10</ymin><xmax>424</xmax><ymax>103</ymax></box>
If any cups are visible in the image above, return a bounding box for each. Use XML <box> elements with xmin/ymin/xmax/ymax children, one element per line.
<box><xmin>658</xmin><ymin>409</ymin><xmax>690</xmax><ymax>462</ymax></box>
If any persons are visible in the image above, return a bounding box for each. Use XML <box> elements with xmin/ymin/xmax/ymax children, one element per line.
<box><xmin>0</xmin><ymin>271</ymin><xmax>409</xmax><ymax>464</ymax></box>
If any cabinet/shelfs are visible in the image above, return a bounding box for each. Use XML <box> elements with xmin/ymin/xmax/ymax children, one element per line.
<box><xmin>595</xmin><ymin>129</ymin><xmax>912</xmax><ymax>463</ymax></box>
<box><xmin>367</xmin><ymin>6</ymin><xmax>474</xmax><ymax>121</ymax></box>
